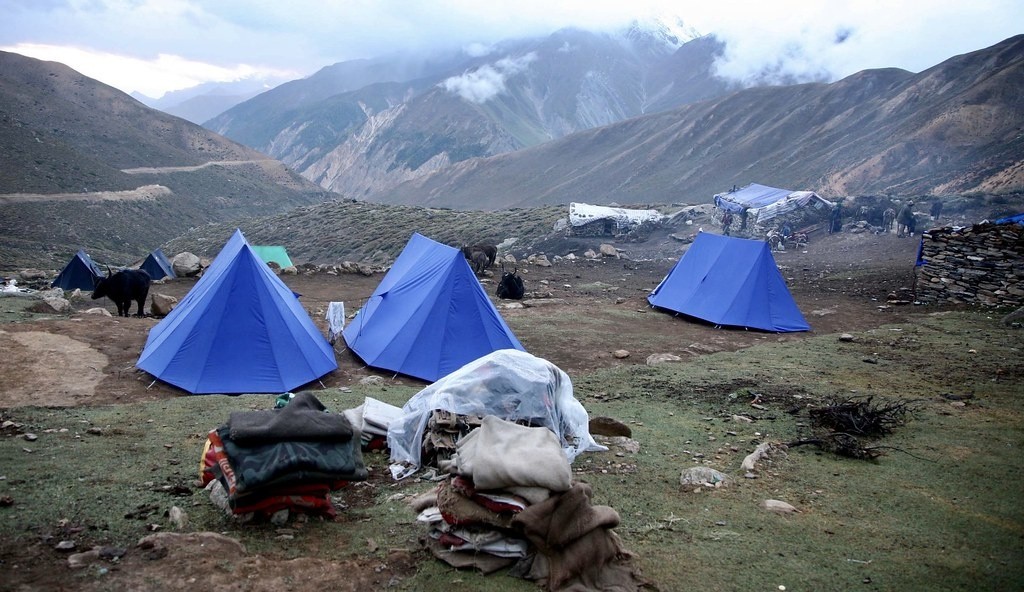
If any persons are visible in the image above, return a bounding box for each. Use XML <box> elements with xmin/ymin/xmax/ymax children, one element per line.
<box><xmin>829</xmin><ymin>208</ymin><xmax>842</xmax><ymax>234</ymax></box>
<box><xmin>897</xmin><ymin>200</ymin><xmax>914</xmax><ymax>238</ymax></box>
<box><xmin>721</xmin><ymin>209</ymin><xmax>733</xmax><ymax>236</ymax></box>
<box><xmin>932</xmin><ymin>198</ymin><xmax>943</xmax><ymax>220</ymax></box>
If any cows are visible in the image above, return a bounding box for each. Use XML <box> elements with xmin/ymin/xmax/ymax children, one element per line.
<box><xmin>929</xmin><ymin>202</ymin><xmax>942</xmax><ymax>219</ymax></box>
<box><xmin>89</xmin><ymin>264</ymin><xmax>151</xmax><ymax>316</ymax></box>
<box><xmin>855</xmin><ymin>205</ymin><xmax>895</xmax><ymax>230</ymax></box>
<box><xmin>461</xmin><ymin>245</ymin><xmax>497</xmax><ymax>275</ymax></box>
<box><xmin>495</xmin><ymin>264</ymin><xmax>524</xmax><ymax>299</ymax></box>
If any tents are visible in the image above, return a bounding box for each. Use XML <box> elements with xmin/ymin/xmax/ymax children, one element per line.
<box><xmin>139</xmin><ymin>248</ymin><xmax>175</xmax><ymax>280</ymax></box>
<box><xmin>136</xmin><ymin>230</ymin><xmax>339</xmax><ymax>393</ymax></box>
<box><xmin>342</xmin><ymin>234</ymin><xmax>526</xmax><ymax>382</ymax></box>
<box><xmin>647</xmin><ymin>231</ymin><xmax>810</xmax><ymax>332</ymax></box>
<box><xmin>251</xmin><ymin>246</ymin><xmax>293</xmax><ymax>270</ymax></box>
<box><xmin>51</xmin><ymin>250</ymin><xmax>104</xmax><ymax>292</ymax></box>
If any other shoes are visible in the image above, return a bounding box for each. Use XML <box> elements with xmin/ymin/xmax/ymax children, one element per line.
<box><xmin>897</xmin><ymin>235</ymin><xmax>904</xmax><ymax>238</ymax></box>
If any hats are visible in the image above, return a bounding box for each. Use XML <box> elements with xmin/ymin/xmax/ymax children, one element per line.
<box><xmin>908</xmin><ymin>200</ymin><xmax>914</xmax><ymax>206</ymax></box>
